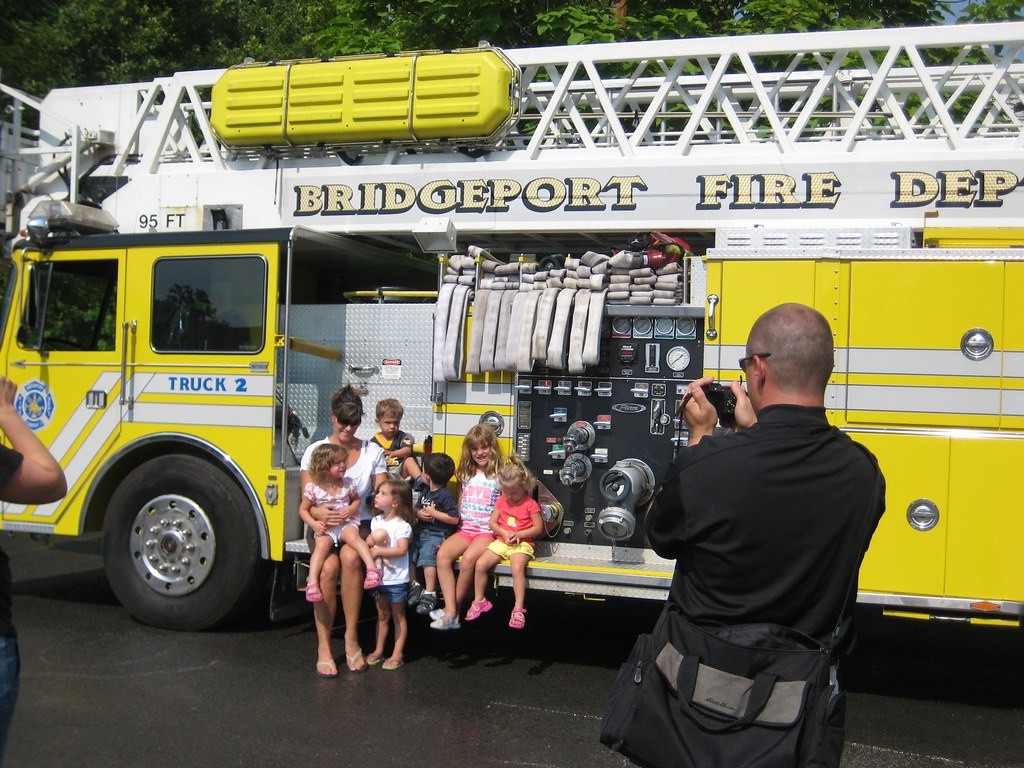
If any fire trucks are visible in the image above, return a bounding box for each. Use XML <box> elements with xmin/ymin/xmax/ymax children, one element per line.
<box><xmin>0</xmin><ymin>25</ymin><xmax>1024</xmax><ymax>633</ymax></box>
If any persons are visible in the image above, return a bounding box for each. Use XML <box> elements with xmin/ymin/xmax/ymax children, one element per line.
<box><xmin>365</xmin><ymin>479</ymin><xmax>412</xmax><ymax>670</ymax></box>
<box><xmin>429</xmin><ymin>423</ymin><xmax>504</xmax><ymax>629</ymax></box>
<box><xmin>370</xmin><ymin>528</ymin><xmax>396</xmax><ymax>570</ymax></box>
<box><xmin>369</xmin><ymin>397</ymin><xmax>426</xmax><ymax>492</ymax></box>
<box><xmin>298</xmin><ymin>444</ymin><xmax>383</xmax><ymax>602</ymax></box>
<box><xmin>409</xmin><ymin>453</ymin><xmax>460</xmax><ymax>614</ymax></box>
<box><xmin>0</xmin><ymin>376</ymin><xmax>68</xmax><ymax>768</ymax></box>
<box><xmin>300</xmin><ymin>383</ymin><xmax>388</xmax><ymax>674</ymax></box>
<box><xmin>646</xmin><ymin>304</ymin><xmax>886</xmax><ymax>768</ymax></box>
<box><xmin>465</xmin><ymin>454</ymin><xmax>543</xmax><ymax>629</ymax></box>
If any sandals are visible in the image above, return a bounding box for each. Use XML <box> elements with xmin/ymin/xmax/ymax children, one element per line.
<box><xmin>430</xmin><ymin>614</ymin><xmax>461</xmax><ymax>630</ymax></box>
<box><xmin>465</xmin><ymin>598</ymin><xmax>492</xmax><ymax>621</ymax></box>
<box><xmin>509</xmin><ymin>608</ymin><xmax>527</xmax><ymax>628</ymax></box>
<box><xmin>364</xmin><ymin>569</ymin><xmax>382</xmax><ymax>589</ymax></box>
<box><xmin>408</xmin><ymin>584</ymin><xmax>422</xmax><ymax>605</ymax></box>
<box><xmin>305</xmin><ymin>583</ymin><xmax>323</xmax><ymax>602</ymax></box>
<box><xmin>430</xmin><ymin>610</ymin><xmax>444</xmax><ymax>621</ymax></box>
<box><xmin>416</xmin><ymin>594</ymin><xmax>438</xmax><ymax>614</ymax></box>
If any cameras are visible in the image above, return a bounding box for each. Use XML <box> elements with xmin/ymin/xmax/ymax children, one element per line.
<box><xmin>701</xmin><ymin>383</ymin><xmax>737</xmax><ymax>421</ymax></box>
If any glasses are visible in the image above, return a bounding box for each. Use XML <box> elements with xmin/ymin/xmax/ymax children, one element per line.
<box><xmin>739</xmin><ymin>353</ymin><xmax>771</xmax><ymax>373</ymax></box>
<box><xmin>338</xmin><ymin>416</ymin><xmax>359</xmax><ymax>426</ymax></box>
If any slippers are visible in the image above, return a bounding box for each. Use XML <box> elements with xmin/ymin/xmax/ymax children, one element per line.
<box><xmin>316</xmin><ymin>658</ymin><xmax>338</xmax><ymax>678</ymax></box>
<box><xmin>382</xmin><ymin>659</ymin><xmax>404</xmax><ymax>670</ymax></box>
<box><xmin>346</xmin><ymin>648</ymin><xmax>367</xmax><ymax>673</ymax></box>
<box><xmin>366</xmin><ymin>653</ymin><xmax>385</xmax><ymax>665</ymax></box>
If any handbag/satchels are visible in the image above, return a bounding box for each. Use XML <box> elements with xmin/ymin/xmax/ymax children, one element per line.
<box><xmin>600</xmin><ymin>602</ymin><xmax>834</xmax><ymax>768</ymax></box>
